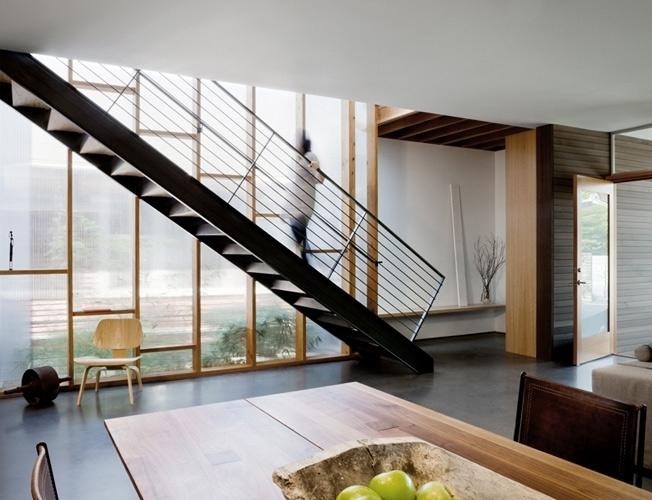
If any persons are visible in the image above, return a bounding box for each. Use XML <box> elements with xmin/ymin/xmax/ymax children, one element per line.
<box><xmin>285</xmin><ymin>128</ymin><xmax>327</xmax><ymax>262</ymax></box>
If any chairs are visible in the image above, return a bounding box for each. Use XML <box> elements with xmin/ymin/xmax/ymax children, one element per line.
<box><xmin>72</xmin><ymin>318</ymin><xmax>146</xmax><ymax>407</ymax></box>
<box><xmin>31</xmin><ymin>439</ymin><xmax>60</xmax><ymax>500</ymax></box>
<box><xmin>511</xmin><ymin>371</ymin><xmax>647</xmax><ymax>490</ymax></box>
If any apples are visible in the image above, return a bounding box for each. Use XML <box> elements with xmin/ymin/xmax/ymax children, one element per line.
<box><xmin>368</xmin><ymin>469</ymin><xmax>415</xmax><ymax>500</ymax></box>
<box><xmin>416</xmin><ymin>481</ymin><xmax>455</xmax><ymax>500</ymax></box>
<box><xmin>336</xmin><ymin>484</ymin><xmax>383</xmax><ymax>500</ymax></box>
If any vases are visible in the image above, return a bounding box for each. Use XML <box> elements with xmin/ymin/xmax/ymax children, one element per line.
<box><xmin>479</xmin><ymin>277</ymin><xmax>494</xmax><ymax>306</ymax></box>
